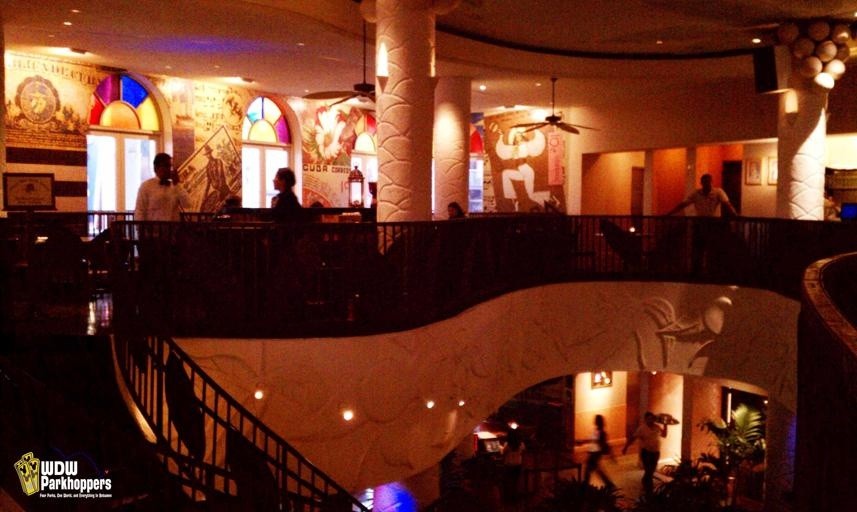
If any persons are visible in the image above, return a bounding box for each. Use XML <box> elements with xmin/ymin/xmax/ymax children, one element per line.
<box><xmin>621</xmin><ymin>412</ymin><xmax>667</xmax><ymax>494</ymax></box>
<box><xmin>500</xmin><ymin>429</ymin><xmax>525</xmax><ymax>506</ymax></box>
<box><xmin>448</xmin><ymin>202</ymin><xmax>469</xmax><ymax>219</ymax></box>
<box><xmin>271</xmin><ymin>168</ymin><xmax>302</xmax><ymax>209</ymax></box>
<box><xmin>134</xmin><ymin>153</ymin><xmax>193</xmax><ymax>223</ymax></box>
<box><xmin>584</xmin><ymin>415</ymin><xmax>616</xmax><ymax>490</ymax></box>
<box><xmin>658</xmin><ymin>175</ymin><xmax>738</xmax><ymax>216</ymax></box>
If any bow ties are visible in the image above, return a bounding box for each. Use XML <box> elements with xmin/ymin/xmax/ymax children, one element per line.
<box><xmin>159</xmin><ymin>179</ymin><xmax>173</xmax><ymax>187</ymax></box>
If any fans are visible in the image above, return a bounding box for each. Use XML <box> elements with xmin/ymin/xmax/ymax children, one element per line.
<box><xmin>300</xmin><ymin>20</ymin><xmax>379</xmax><ymax>106</ymax></box>
<box><xmin>510</xmin><ymin>76</ymin><xmax>597</xmax><ymax>136</ymax></box>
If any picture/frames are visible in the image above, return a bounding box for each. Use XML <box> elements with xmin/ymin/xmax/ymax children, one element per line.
<box><xmin>591</xmin><ymin>372</ymin><xmax>612</xmax><ymax>388</ymax></box>
<box><xmin>4</xmin><ymin>171</ymin><xmax>57</xmax><ymax>211</ymax></box>
<box><xmin>745</xmin><ymin>156</ymin><xmax>777</xmax><ymax>186</ymax></box>
